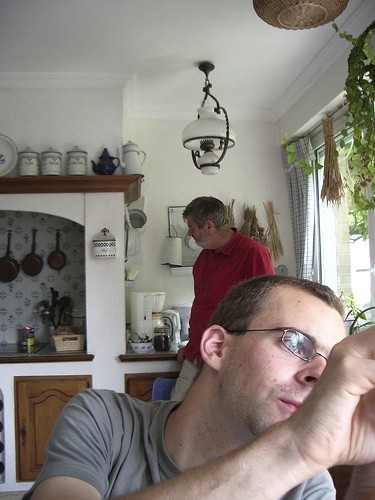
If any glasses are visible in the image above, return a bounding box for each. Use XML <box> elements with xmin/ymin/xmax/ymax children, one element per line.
<box><xmin>225</xmin><ymin>327</ymin><xmax>329</xmax><ymax>363</ymax></box>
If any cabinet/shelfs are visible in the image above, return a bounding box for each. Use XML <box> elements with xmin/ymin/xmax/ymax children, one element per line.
<box><xmin>13</xmin><ymin>375</ymin><xmax>92</xmax><ymax>483</ymax></box>
<box><xmin>125</xmin><ymin>370</ymin><xmax>181</xmax><ymax>402</ymax></box>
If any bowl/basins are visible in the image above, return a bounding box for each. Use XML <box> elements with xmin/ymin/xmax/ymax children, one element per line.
<box><xmin>128</xmin><ymin>342</ymin><xmax>153</xmax><ymax>355</ymax></box>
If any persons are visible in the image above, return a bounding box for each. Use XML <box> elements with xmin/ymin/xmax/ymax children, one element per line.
<box><xmin>171</xmin><ymin>196</ymin><xmax>277</xmax><ymax>400</ymax></box>
<box><xmin>22</xmin><ymin>274</ymin><xmax>375</xmax><ymax>500</ymax></box>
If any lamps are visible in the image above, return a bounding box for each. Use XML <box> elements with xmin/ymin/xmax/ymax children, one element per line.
<box><xmin>182</xmin><ymin>62</ymin><xmax>236</xmax><ymax>174</ymax></box>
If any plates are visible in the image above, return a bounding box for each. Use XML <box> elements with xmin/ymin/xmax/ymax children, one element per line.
<box><xmin>0</xmin><ymin>134</ymin><xmax>18</xmax><ymax>178</ymax></box>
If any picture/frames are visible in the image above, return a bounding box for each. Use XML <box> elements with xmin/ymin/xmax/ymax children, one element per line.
<box><xmin>168</xmin><ymin>206</ymin><xmax>203</xmax><ymax>268</ymax></box>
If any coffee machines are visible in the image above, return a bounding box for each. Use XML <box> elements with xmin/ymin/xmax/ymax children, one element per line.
<box><xmin>130</xmin><ymin>292</ymin><xmax>163</xmax><ymax>342</ymax></box>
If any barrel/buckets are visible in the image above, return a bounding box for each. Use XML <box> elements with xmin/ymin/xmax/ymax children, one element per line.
<box><xmin>18</xmin><ymin>147</ymin><xmax>88</xmax><ymax>176</ymax></box>
<box><xmin>48</xmin><ymin>324</ymin><xmax>71</xmax><ymax>350</ymax></box>
<box><xmin>17</xmin><ymin>328</ymin><xmax>35</xmax><ymax>354</ymax></box>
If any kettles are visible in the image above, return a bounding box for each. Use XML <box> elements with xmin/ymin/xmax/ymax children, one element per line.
<box><xmin>162</xmin><ymin>310</ymin><xmax>181</xmax><ymax>351</ymax></box>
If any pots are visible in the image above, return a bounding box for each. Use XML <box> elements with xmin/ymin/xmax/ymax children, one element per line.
<box><xmin>22</xmin><ymin>230</ymin><xmax>43</xmax><ymax>276</ymax></box>
<box><xmin>0</xmin><ymin>233</ymin><xmax>19</xmax><ymax>283</ymax></box>
<box><xmin>47</xmin><ymin>232</ymin><xmax>66</xmax><ymax>270</ymax></box>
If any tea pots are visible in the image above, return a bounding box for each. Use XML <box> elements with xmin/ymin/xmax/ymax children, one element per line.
<box><xmin>91</xmin><ymin>141</ymin><xmax>146</xmax><ymax>178</ymax></box>
